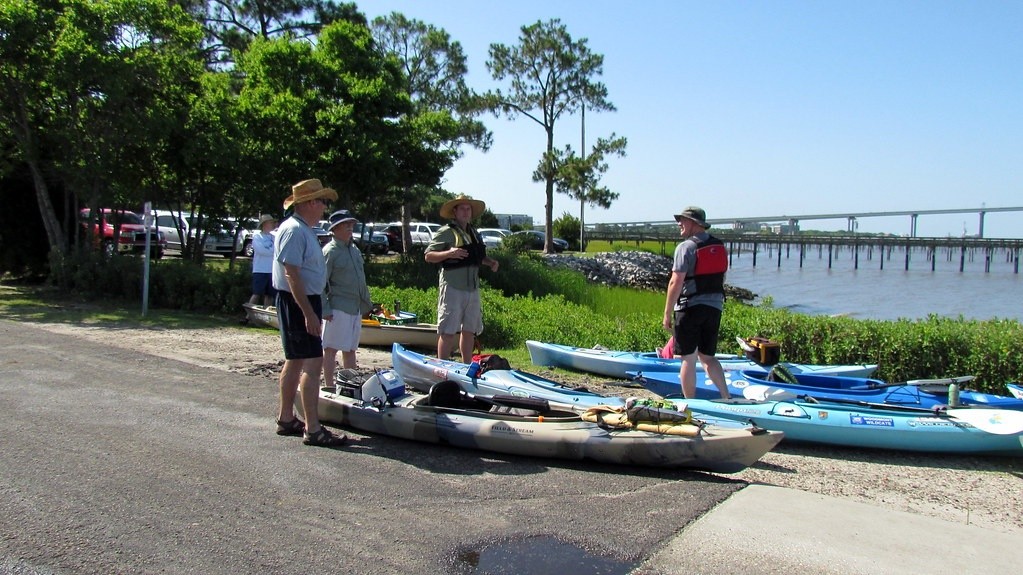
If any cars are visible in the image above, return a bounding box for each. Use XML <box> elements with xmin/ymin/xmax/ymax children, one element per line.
<box><xmin>79</xmin><ymin>208</ymin><xmax>167</xmax><ymax>260</ymax></box>
<box><xmin>142</xmin><ymin>210</ymin><xmax>569</xmax><ymax>259</ymax></box>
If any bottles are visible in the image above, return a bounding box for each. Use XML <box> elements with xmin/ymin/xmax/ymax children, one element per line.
<box><xmin>393</xmin><ymin>299</ymin><xmax>401</xmax><ymax>316</ymax></box>
<box><xmin>948</xmin><ymin>379</ymin><xmax>959</xmax><ymax>407</ymax></box>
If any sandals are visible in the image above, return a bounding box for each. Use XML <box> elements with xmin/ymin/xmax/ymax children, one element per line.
<box><xmin>304</xmin><ymin>426</ymin><xmax>347</xmax><ymax>446</ymax></box>
<box><xmin>276</xmin><ymin>417</ymin><xmax>305</xmax><ymax>436</ymax></box>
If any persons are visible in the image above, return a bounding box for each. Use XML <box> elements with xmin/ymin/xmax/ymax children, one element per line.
<box><xmin>271</xmin><ymin>178</ymin><xmax>348</xmax><ymax>447</ymax></box>
<box><xmin>240</xmin><ymin>213</ymin><xmax>276</xmax><ymax>325</ymax></box>
<box><xmin>321</xmin><ymin>209</ymin><xmax>373</xmax><ymax>386</ymax></box>
<box><xmin>662</xmin><ymin>207</ymin><xmax>731</xmax><ymax>399</ymax></box>
<box><xmin>423</xmin><ymin>195</ymin><xmax>499</xmax><ymax>365</ymax></box>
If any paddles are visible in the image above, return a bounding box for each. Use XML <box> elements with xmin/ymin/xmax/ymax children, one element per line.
<box><xmin>742</xmin><ymin>384</ymin><xmax>1023</xmax><ymax>435</ymax></box>
<box><xmin>673</xmin><ymin>353</ymin><xmax>746</xmax><ymax>360</ymax></box>
<box><xmin>849</xmin><ymin>375</ymin><xmax>975</xmax><ymax>389</ymax></box>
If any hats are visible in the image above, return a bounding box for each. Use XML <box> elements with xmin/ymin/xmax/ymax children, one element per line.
<box><xmin>257</xmin><ymin>214</ymin><xmax>279</xmax><ymax>230</ymax></box>
<box><xmin>328</xmin><ymin>210</ymin><xmax>359</xmax><ymax>230</ymax></box>
<box><xmin>674</xmin><ymin>207</ymin><xmax>711</xmax><ymax>230</ymax></box>
<box><xmin>283</xmin><ymin>179</ymin><xmax>338</xmax><ymax>210</ymax></box>
<box><xmin>440</xmin><ymin>194</ymin><xmax>486</xmax><ymax>219</ymax></box>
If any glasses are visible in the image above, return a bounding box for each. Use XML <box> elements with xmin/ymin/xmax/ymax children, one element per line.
<box><xmin>316</xmin><ymin>198</ymin><xmax>329</xmax><ymax>205</ymax></box>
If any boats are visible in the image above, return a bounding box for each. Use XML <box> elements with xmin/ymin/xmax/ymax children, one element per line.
<box><xmin>624</xmin><ymin>364</ymin><xmax>1023</xmax><ymax>411</ymax></box>
<box><xmin>526</xmin><ymin>334</ymin><xmax>877</xmax><ymax>381</ymax></box>
<box><xmin>293</xmin><ymin>368</ymin><xmax>784</xmax><ymax>474</ymax></box>
<box><xmin>664</xmin><ymin>399</ymin><xmax>1023</xmax><ymax>452</ymax></box>
<box><xmin>392</xmin><ymin>343</ymin><xmax>755</xmax><ymax>428</ymax></box>
<box><xmin>242</xmin><ymin>300</ymin><xmax>475</xmax><ymax>353</ymax></box>
